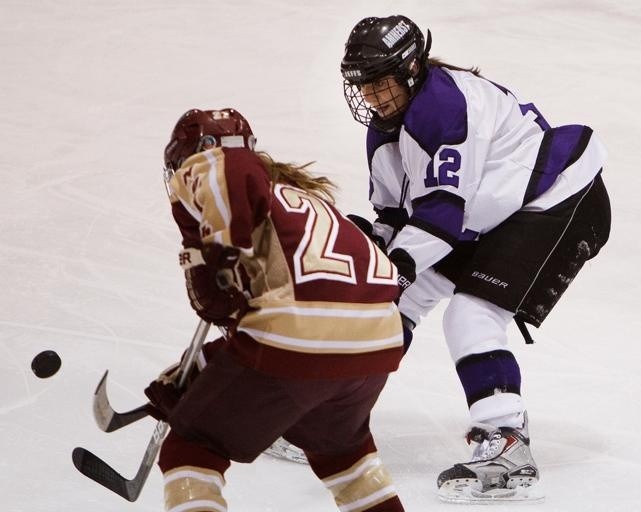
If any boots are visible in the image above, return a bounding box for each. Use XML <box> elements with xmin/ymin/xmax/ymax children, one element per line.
<box><xmin>436</xmin><ymin>411</ymin><xmax>539</xmax><ymax>487</ymax></box>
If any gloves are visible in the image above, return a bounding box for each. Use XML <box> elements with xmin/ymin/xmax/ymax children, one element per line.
<box><xmin>144</xmin><ymin>348</ymin><xmax>207</xmax><ymax>422</ymax></box>
<box><xmin>183</xmin><ymin>244</ymin><xmax>250</xmax><ymax>326</ymax></box>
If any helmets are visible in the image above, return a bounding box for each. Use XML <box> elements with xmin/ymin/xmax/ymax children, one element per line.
<box><xmin>163</xmin><ymin>109</ymin><xmax>257</xmax><ymax>202</ymax></box>
<box><xmin>341</xmin><ymin>15</ymin><xmax>431</xmax><ymax>138</ymax></box>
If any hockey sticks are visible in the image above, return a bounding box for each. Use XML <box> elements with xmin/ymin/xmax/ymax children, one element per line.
<box><xmin>72</xmin><ymin>320</ymin><xmax>209</xmax><ymax>502</ymax></box>
<box><xmin>93</xmin><ymin>370</ymin><xmax>157</xmax><ymax>433</ymax></box>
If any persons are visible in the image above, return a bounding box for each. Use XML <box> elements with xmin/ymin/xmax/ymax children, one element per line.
<box><xmin>345</xmin><ymin>15</ymin><xmax>611</xmax><ymax>503</ymax></box>
<box><xmin>144</xmin><ymin>108</ymin><xmax>405</xmax><ymax>511</ymax></box>
